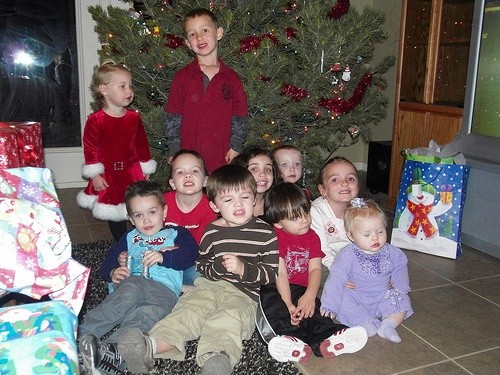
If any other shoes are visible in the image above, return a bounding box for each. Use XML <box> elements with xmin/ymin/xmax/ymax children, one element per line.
<box><xmin>117</xmin><ymin>328</ymin><xmax>152</xmax><ymax>374</ymax></box>
<box><xmin>198</xmin><ymin>352</ymin><xmax>232</xmax><ymax>375</ymax></box>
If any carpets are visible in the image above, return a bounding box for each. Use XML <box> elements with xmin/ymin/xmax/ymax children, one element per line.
<box><xmin>72</xmin><ymin>239</ymin><xmax>303</xmax><ymax>375</ymax></box>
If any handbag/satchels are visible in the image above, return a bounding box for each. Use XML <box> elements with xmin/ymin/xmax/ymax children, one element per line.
<box><xmin>390</xmin><ymin>161</ymin><xmax>470</xmax><ymax>259</ymax></box>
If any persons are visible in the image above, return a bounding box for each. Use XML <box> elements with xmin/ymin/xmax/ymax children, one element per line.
<box><xmin>78</xmin><ymin>144</ymin><xmax>414</xmax><ymax>375</ymax></box>
<box><xmin>77</xmin><ymin>65</ymin><xmax>157</xmax><ymax>242</ymax></box>
<box><xmin>166</xmin><ymin>7</ymin><xmax>247</xmax><ymax>192</ymax></box>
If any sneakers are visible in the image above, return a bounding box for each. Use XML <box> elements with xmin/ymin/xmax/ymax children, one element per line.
<box><xmin>79</xmin><ymin>333</ymin><xmax>119</xmax><ymax>375</ymax></box>
<box><xmin>269</xmin><ymin>335</ymin><xmax>312</xmax><ymax>363</ymax></box>
<box><xmin>320</xmin><ymin>326</ymin><xmax>367</xmax><ymax>358</ymax></box>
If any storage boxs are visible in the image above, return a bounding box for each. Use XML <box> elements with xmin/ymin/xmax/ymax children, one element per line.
<box><xmin>0</xmin><ymin>122</ymin><xmax>45</xmax><ymax>168</ymax></box>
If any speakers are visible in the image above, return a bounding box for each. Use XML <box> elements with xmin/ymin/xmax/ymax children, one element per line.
<box><xmin>366</xmin><ymin>140</ymin><xmax>390</xmax><ymax>195</ymax></box>
